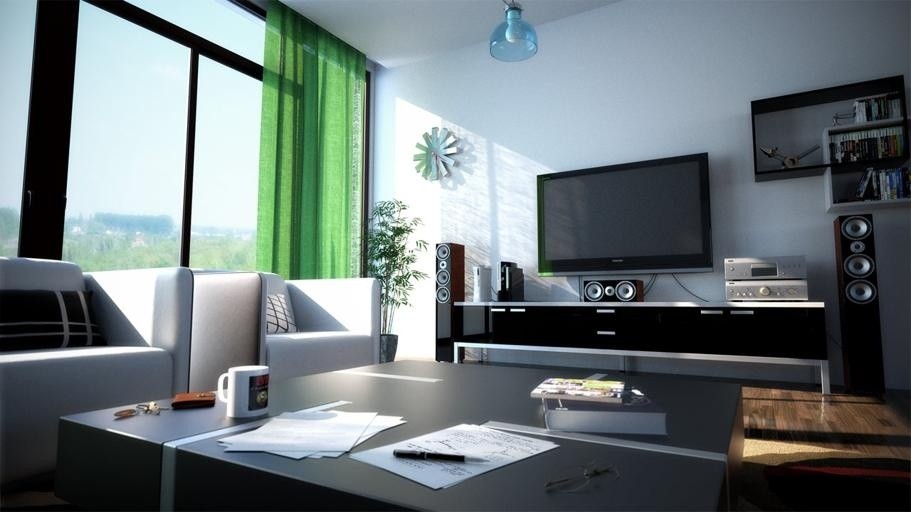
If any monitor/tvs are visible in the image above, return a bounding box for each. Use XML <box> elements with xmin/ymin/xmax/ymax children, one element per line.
<box><xmin>536</xmin><ymin>151</ymin><xmax>715</xmax><ymax>275</ymax></box>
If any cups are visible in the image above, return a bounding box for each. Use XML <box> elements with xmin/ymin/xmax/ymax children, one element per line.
<box><xmin>216</xmin><ymin>364</ymin><xmax>271</xmax><ymax>419</ymax></box>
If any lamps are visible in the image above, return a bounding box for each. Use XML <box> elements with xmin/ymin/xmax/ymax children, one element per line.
<box><xmin>490</xmin><ymin>0</ymin><xmax>537</xmax><ymax>62</ymax></box>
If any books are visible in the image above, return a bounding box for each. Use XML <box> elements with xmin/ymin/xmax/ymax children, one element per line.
<box><xmin>542</xmin><ymin>385</ymin><xmax>671</xmax><ymax>436</ymax></box>
<box><xmin>852</xmin><ymin>166</ymin><xmax>906</xmax><ymax>200</ymax></box>
<box><xmin>530</xmin><ymin>377</ymin><xmax>625</xmax><ymax>405</ymax></box>
<box><xmin>827</xmin><ymin>126</ymin><xmax>904</xmax><ymax>165</ymax></box>
<box><xmin>852</xmin><ymin>95</ymin><xmax>903</xmax><ymax>124</ymax></box>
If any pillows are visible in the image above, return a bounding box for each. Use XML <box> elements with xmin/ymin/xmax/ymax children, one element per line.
<box><xmin>1</xmin><ymin>289</ymin><xmax>108</xmax><ymax>352</ymax></box>
<box><xmin>265</xmin><ymin>293</ymin><xmax>298</xmax><ymax>333</ymax></box>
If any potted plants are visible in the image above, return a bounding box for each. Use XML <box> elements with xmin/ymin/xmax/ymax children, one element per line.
<box><xmin>360</xmin><ymin>198</ymin><xmax>431</xmax><ymax>364</ymax></box>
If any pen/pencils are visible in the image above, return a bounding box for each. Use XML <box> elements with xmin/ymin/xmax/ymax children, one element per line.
<box><xmin>394</xmin><ymin>447</ymin><xmax>489</xmax><ymax>464</ymax></box>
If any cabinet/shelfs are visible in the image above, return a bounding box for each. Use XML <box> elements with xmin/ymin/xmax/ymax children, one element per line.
<box><xmin>453</xmin><ymin>300</ymin><xmax>833</xmax><ymax>397</ymax></box>
<box><xmin>751</xmin><ymin>75</ymin><xmax>911</xmax><ymax>212</ymax></box>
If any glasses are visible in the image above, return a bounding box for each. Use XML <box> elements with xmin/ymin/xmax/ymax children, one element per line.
<box><xmin>543</xmin><ymin>464</ymin><xmax>622</xmax><ymax>493</ymax></box>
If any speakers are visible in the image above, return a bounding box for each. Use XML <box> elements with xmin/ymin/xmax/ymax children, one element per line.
<box><xmin>832</xmin><ymin>213</ymin><xmax>888</xmax><ymax>399</ymax></box>
<box><xmin>581</xmin><ymin>277</ymin><xmax>644</xmax><ymax>302</ymax></box>
<box><xmin>433</xmin><ymin>242</ymin><xmax>465</xmax><ymax>363</ymax></box>
<box><xmin>497</xmin><ymin>261</ymin><xmax>517</xmax><ymax>302</ymax></box>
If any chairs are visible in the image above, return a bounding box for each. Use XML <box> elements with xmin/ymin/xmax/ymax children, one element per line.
<box><xmin>189</xmin><ymin>268</ymin><xmax>382</xmax><ymax>396</ymax></box>
<box><xmin>0</xmin><ymin>258</ymin><xmax>194</xmax><ymax>485</ymax></box>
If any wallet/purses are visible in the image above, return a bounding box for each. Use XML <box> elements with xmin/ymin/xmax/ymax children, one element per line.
<box><xmin>171</xmin><ymin>392</ymin><xmax>215</xmax><ymax>410</ymax></box>
